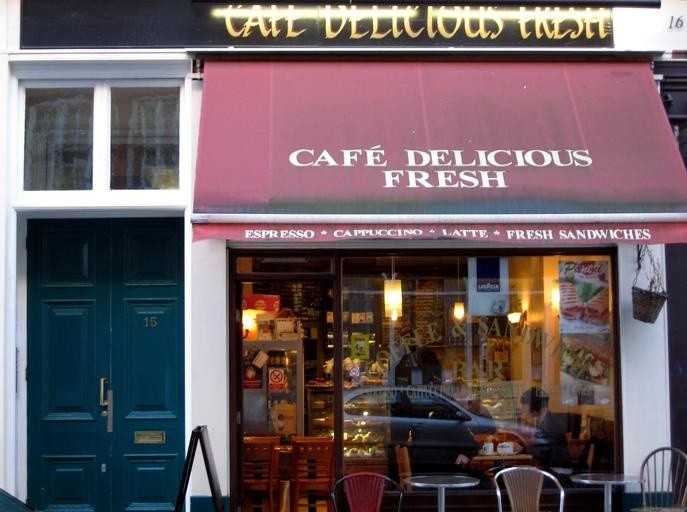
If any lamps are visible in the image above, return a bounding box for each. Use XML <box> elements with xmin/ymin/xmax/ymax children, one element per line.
<box><xmin>384</xmin><ymin>257</ymin><xmax>403</xmax><ymax>321</ymax></box>
<box><xmin>242</xmin><ymin>309</ymin><xmax>266</xmax><ymax>339</ymax></box>
<box><xmin>454</xmin><ymin>256</ymin><xmax>465</xmax><ymax>319</ymax></box>
<box><xmin>506</xmin><ymin>298</ymin><xmax>525</xmax><ymax>323</ymax></box>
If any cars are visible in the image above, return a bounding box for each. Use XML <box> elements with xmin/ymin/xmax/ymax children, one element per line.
<box><xmin>325</xmin><ymin>381</ymin><xmax>551</xmax><ymax>465</ymax></box>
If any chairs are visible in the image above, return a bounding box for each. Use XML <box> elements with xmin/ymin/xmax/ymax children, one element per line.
<box><xmin>394</xmin><ymin>445</ymin><xmax>469</xmax><ymax>491</ymax></box>
<box><xmin>243</xmin><ymin>435</ymin><xmax>335</xmax><ymax>512</ymax></box>
<box><xmin>629</xmin><ymin>448</ymin><xmax>687</xmax><ymax>512</ymax></box>
<box><xmin>330</xmin><ymin>473</ymin><xmax>403</xmax><ymax>512</ymax></box>
<box><xmin>538</xmin><ymin>443</ymin><xmax>594</xmax><ymax>489</ymax></box>
<box><xmin>494</xmin><ymin>467</ymin><xmax>565</xmax><ymax>512</ymax></box>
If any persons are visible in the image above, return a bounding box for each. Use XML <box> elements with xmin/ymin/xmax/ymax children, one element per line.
<box><xmin>390</xmin><ymin>384</ymin><xmax>479</xmax><ymax>493</ymax></box>
<box><xmin>395</xmin><ymin>330</ymin><xmax>442</xmax><ymax>387</ymax></box>
<box><xmin>520</xmin><ymin>387</ymin><xmax>574</xmax><ymax>488</ymax></box>
<box><xmin>450</xmin><ymin>379</ymin><xmax>497</xmax><ymax>434</ymax></box>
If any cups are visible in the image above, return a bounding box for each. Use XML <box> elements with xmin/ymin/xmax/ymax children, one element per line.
<box><xmin>482</xmin><ymin>441</ymin><xmax>514</xmax><ymax>454</ymax></box>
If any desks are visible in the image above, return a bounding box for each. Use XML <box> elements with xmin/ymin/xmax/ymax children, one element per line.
<box><xmin>472</xmin><ymin>455</ymin><xmax>533</xmax><ymax>489</ymax></box>
<box><xmin>570</xmin><ymin>473</ymin><xmax>644</xmax><ymax>511</ymax></box>
<box><xmin>403</xmin><ymin>476</ymin><xmax>480</xmax><ymax>512</ymax></box>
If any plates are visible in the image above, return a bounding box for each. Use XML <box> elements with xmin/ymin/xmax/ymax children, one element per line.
<box><xmin>478</xmin><ymin>451</ymin><xmax>519</xmax><ymax>456</ymax></box>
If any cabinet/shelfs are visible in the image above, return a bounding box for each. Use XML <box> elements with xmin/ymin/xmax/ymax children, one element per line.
<box><xmin>302</xmin><ymin>322</ymin><xmax>325</xmax><ymax>389</ymax></box>
<box><xmin>242</xmin><ymin>340</ymin><xmax>304</xmax><ymax>445</ymax></box>
<box><xmin>305</xmin><ymin>379</ymin><xmax>535</xmax><ymax>466</ymax></box>
<box><xmin>326</xmin><ymin>288</ymin><xmax>380</xmax><ymax>376</ymax></box>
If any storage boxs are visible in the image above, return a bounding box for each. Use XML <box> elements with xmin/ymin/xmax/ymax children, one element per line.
<box><xmin>274</xmin><ymin>317</ymin><xmax>294</xmax><ymax>333</ymax></box>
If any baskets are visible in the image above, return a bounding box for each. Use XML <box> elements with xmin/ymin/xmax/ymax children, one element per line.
<box><xmin>631</xmin><ymin>286</ymin><xmax>667</xmax><ymax>324</ymax></box>
